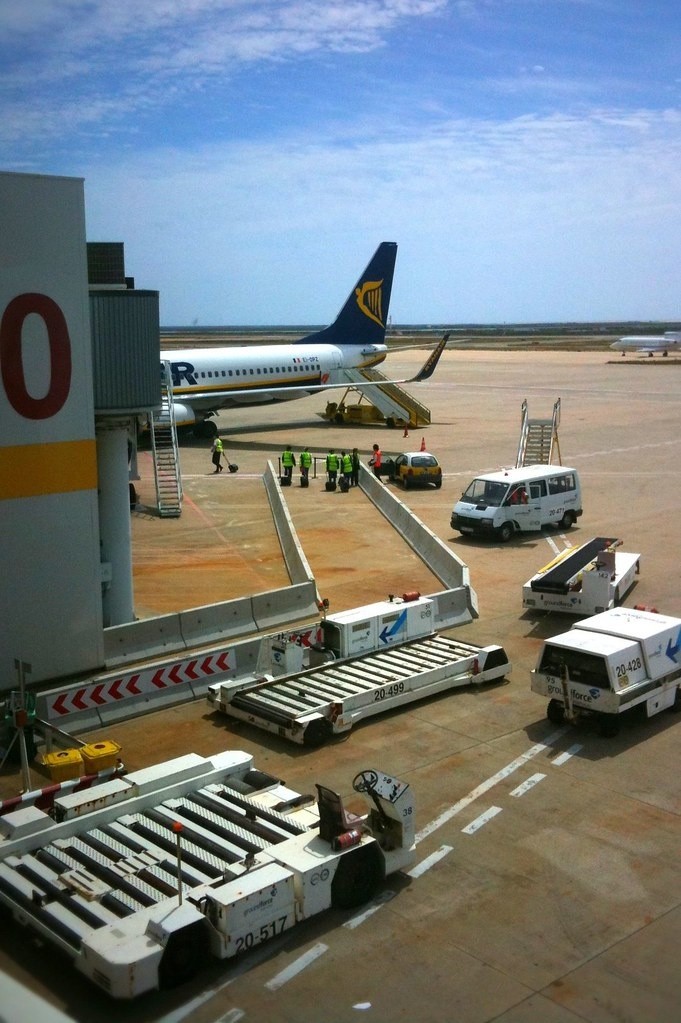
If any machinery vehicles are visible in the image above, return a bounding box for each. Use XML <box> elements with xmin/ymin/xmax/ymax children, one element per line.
<box><xmin>1</xmin><ymin>752</ymin><xmax>418</xmax><ymax>1003</ymax></box>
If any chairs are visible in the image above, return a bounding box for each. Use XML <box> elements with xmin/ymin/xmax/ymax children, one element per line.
<box><xmin>310</xmin><ymin>621</ymin><xmax>333</xmax><ymax>652</ymax></box>
<box><xmin>314</xmin><ymin>783</ymin><xmax>363</xmax><ymax>843</ymax></box>
<box><xmin>596</xmin><ymin>547</ymin><xmax>615</xmax><ymax>582</ymax></box>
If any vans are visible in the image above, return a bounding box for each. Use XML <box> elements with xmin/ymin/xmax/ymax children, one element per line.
<box><xmin>448</xmin><ymin>463</ymin><xmax>581</xmax><ymax>541</ymax></box>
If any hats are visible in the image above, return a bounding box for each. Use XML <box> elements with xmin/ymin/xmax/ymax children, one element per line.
<box><xmin>516</xmin><ymin>484</ymin><xmax>524</xmax><ymax>488</ymax></box>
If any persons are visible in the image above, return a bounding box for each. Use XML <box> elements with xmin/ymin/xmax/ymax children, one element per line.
<box><xmin>282</xmin><ymin>445</ymin><xmax>296</xmax><ymax>483</ymax></box>
<box><xmin>369</xmin><ymin>444</ymin><xmax>382</xmax><ymax>481</ymax></box>
<box><xmin>299</xmin><ymin>447</ymin><xmax>312</xmax><ymax>480</ymax></box>
<box><xmin>326</xmin><ymin>449</ymin><xmax>339</xmax><ymax>483</ymax></box>
<box><xmin>340</xmin><ymin>450</ymin><xmax>353</xmax><ymax>484</ymax></box>
<box><xmin>516</xmin><ymin>484</ymin><xmax>529</xmax><ymax>505</ymax></box>
<box><xmin>349</xmin><ymin>448</ymin><xmax>361</xmax><ymax>486</ymax></box>
<box><xmin>211</xmin><ymin>434</ymin><xmax>225</xmax><ymax>473</ymax></box>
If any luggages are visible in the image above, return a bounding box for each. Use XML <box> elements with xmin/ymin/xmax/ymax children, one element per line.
<box><xmin>301</xmin><ymin>473</ymin><xmax>308</xmax><ymax>487</ymax></box>
<box><xmin>281</xmin><ymin>476</ymin><xmax>291</xmax><ymax>485</ymax></box>
<box><xmin>338</xmin><ymin>473</ymin><xmax>349</xmax><ymax>492</ymax></box>
<box><xmin>326</xmin><ymin>471</ymin><xmax>336</xmax><ymax>491</ymax></box>
<box><xmin>222</xmin><ymin>452</ymin><xmax>238</xmax><ymax>472</ymax></box>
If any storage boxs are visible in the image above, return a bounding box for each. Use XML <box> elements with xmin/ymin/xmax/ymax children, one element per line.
<box><xmin>40</xmin><ymin>740</ymin><xmax>122</xmax><ymax>783</ymax></box>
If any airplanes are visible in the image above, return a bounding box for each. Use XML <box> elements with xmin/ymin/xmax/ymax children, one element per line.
<box><xmin>609</xmin><ymin>330</ymin><xmax>681</xmax><ymax>357</ymax></box>
<box><xmin>137</xmin><ymin>237</ymin><xmax>434</xmax><ymax>429</ymax></box>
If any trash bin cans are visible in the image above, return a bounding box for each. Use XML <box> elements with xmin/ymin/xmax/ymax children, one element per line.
<box><xmin>79</xmin><ymin>739</ymin><xmax>122</xmax><ymax>775</ymax></box>
<box><xmin>41</xmin><ymin>747</ymin><xmax>84</xmax><ymax>784</ymax></box>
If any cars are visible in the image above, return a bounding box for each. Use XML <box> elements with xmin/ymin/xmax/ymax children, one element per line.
<box><xmin>371</xmin><ymin>452</ymin><xmax>443</xmax><ymax>490</ymax></box>
